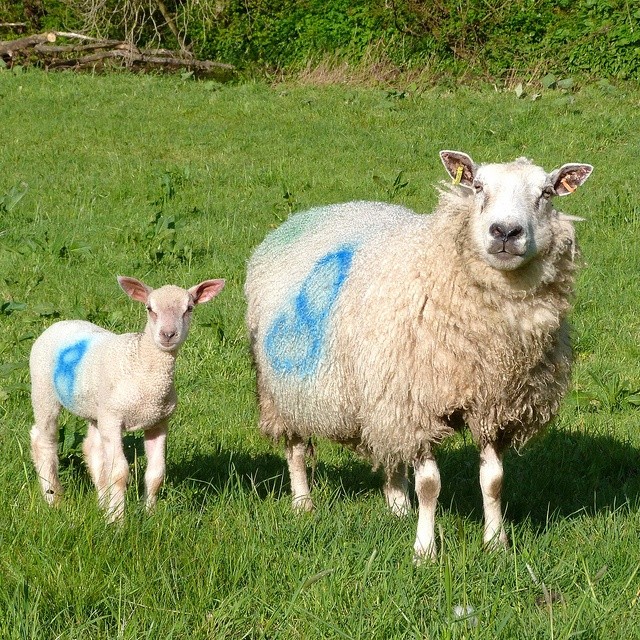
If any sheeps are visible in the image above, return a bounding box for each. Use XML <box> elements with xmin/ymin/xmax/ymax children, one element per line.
<box><xmin>244</xmin><ymin>149</ymin><xmax>594</xmax><ymax>565</ymax></box>
<box><xmin>29</xmin><ymin>274</ymin><xmax>225</xmax><ymax>525</ymax></box>
